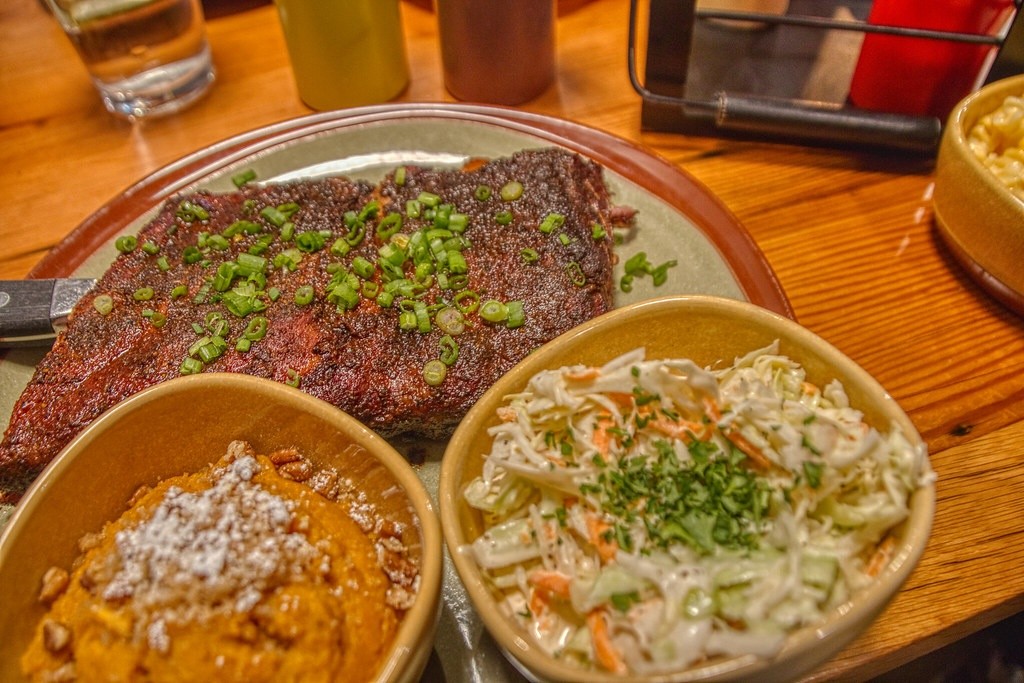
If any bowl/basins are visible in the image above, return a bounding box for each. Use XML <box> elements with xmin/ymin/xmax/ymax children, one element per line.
<box><xmin>439</xmin><ymin>294</ymin><xmax>935</xmax><ymax>683</ymax></box>
<box><xmin>2</xmin><ymin>374</ymin><xmax>447</xmax><ymax>681</ymax></box>
<box><xmin>933</xmin><ymin>75</ymin><xmax>1024</xmax><ymax>315</ymax></box>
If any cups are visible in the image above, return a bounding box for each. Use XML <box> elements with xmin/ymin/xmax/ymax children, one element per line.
<box><xmin>45</xmin><ymin>0</ymin><xmax>218</xmax><ymax>116</ymax></box>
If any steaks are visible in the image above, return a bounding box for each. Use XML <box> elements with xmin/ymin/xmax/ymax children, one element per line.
<box><xmin>0</xmin><ymin>145</ymin><xmax>619</xmax><ymax>508</ymax></box>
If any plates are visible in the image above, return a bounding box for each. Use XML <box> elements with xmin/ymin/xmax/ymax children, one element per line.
<box><xmin>1</xmin><ymin>101</ymin><xmax>797</xmax><ymax>521</ymax></box>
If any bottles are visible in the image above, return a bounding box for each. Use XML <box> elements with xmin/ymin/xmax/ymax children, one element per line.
<box><xmin>276</xmin><ymin>0</ymin><xmax>410</xmax><ymax>110</ymax></box>
<box><xmin>844</xmin><ymin>0</ymin><xmax>1013</xmax><ymax>125</ymax></box>
<box><xmin>435</xmin><ymin>0</ymin><xmax>556</xmax><ymax>104</ymax></box>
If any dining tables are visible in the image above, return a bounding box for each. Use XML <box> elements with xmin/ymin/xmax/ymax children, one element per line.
<box><xmin>1</xmin><ymin>0</ymin><xmax>1024</xmax><ymax>682</ymax></box>
